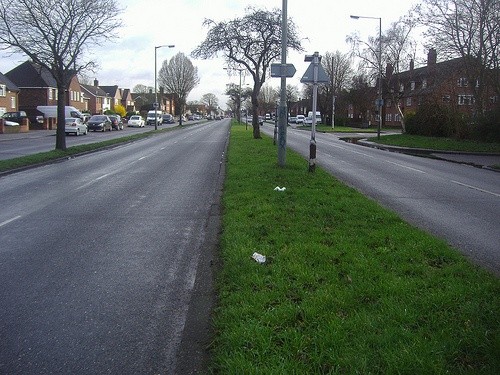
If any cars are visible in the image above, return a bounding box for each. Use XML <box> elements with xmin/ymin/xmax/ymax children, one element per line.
<box><xmin>87</xmin><ymin>115</ymin><xmax>113</xmax><ymax>132</ymax></box>
<box><xmin>128</xmin><ymin>115</ymin><xmax>145</xmax><ymax>128</ymax></box>
<box><xmin>65</xmin><ymin>118</ymin><xmax>88</xmax><ymax>135</ymax></box>
<box><xmin>248</xmin><ymin>113</ymin><xmax>306</xmax><ymax>127</ymax></box>
<box><xmin>177</xmin><ymin>110</ymin><xmax>224</xmax><ymax>123</ymax></box>
<box><xmin>163</xmin><ymin>114</ymin><xmax>175</xmax><ymax>124</ymax></box>
<box><xmin>108</xmin><ymin>114</ymin><xmax>124</xmax><ymax>130</ymax></box>
<box><xmin>303</xmin><ymin>117</ymin><xmax>317</xmax><ymax>127</ymax></box>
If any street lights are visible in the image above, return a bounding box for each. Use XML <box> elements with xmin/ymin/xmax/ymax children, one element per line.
<box><xmin>350</xmin><ymin>15</ymin><xmax>383</xmax><ymax>143</ymax></box>
<box><xmin>223</xmin><ymin>68</ymin><xmax>242</xmax><ymax>124</ymax></box>
<box><xmin>154</xmin><ymin>44</ymin><xmax>176</xmax><ymax>131</ymax></box>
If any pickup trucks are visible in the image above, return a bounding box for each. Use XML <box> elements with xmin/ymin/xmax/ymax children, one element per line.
<box><xmin>1</xmin><ymin>111</ymin><xmax>44</xmax><ymax>130</ymax></box>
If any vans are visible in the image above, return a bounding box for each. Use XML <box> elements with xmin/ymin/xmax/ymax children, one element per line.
<box><xmin>308</xmin><ymin>111</ymin><xmax>322</xmax><ymax>124</ymax></box>
<box><xmin>36</xmin><ymin>105</ymin><xmax>85</xmax><ymax>125</ymax></box>
<box><xmin>146</xmin><ymin>110</ymin><xmax>163</xmax><ymax>126</ymax></box>
